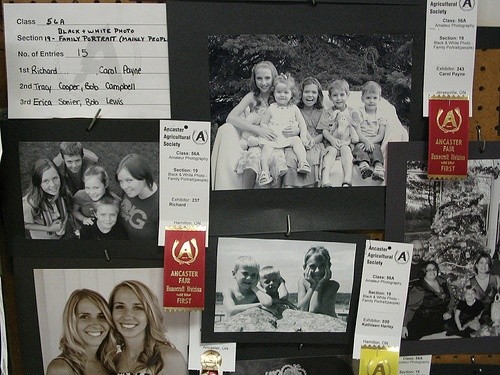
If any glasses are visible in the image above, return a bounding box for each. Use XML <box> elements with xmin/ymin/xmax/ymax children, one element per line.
<box><xmin>425</xmin><ymin>268</ymin><xmax>436</xmax><ymax>275</ymax></box>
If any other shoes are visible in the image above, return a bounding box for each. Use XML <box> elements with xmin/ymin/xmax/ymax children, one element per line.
<box><xmin>371</xmin><ymin>166</ymin><xmax>385</xmax><ymax>182</ymax></box>
<box><xmin>359</xmin><ymin>162</ymin><xmax>373</xmax><ymax>179</ymax></box>
<box><xmin>342</xmin><ymin>179</ymin><xmax>352</xmax><ymax>187</ymax></box>
<box><xmin>320</xmin><ymin>183</ymin><xmax>331</xmax><ymax>188</ymax></box>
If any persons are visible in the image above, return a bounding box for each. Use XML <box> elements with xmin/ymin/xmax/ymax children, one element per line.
<box><xmin>100</xmin><ymin>280</ymin><xmax>188</xmax><ymax>375</ymax></box>
<box><xmin>22</xmin><ymin>142</ymin><xmax>159</xmax><ymax>239</ymax></box>
<box><xmin>256</xmin><ymin>266</ymin><xmax>289</xmax><ymax>319</ymax></box>
<box><xmin>298</xmin><ymin>247</ymin><xmax>339</xmax><ymax>319</ymax></box>
<box><xmin>402</xmin><ymin>240</ymin><xmax>500</xmax><ymax>340</ymax></box>
<box><xmin>223</xmin><ymin>256</ymin><xmax>272</xmax><ymax>320</ymax></box>
<box><xmin>226</xmin><ymin>61</ymin><xmax>387</xmax><ymax>189</ymax></box>
<box><xmin>46</xmin><ymin>289</ymin><xmax>118</xmax><ymax>375</ymax></box>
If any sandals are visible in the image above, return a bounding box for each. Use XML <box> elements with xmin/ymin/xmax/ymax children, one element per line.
<box><xmin>278</xmin><ymin>162</ymin><xmax>288</xmax><ymax>176</ymax></box>
<box><xmin>296</xmin><ymin>162</ymin><xmax>311</xmax><ymax>173</ymax></box>
<box><xmin>259</xmin><ymin>171</ymin><xmax>274</xmax><ymax>185</ymax></box>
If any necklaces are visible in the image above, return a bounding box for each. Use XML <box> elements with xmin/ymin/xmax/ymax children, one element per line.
<box><xmin>126</xmin><ymin>350</ymin><xmax>138</xmax><ymax>373</ymax></box>
